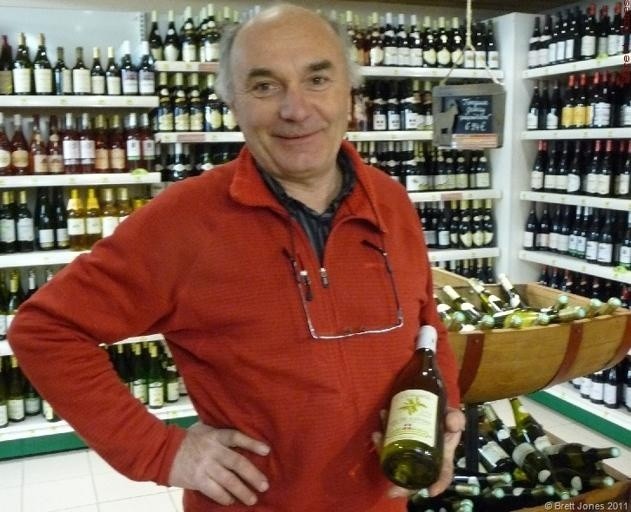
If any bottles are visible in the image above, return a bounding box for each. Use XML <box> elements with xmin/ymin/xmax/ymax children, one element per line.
<box><xmin>152</xmin><ymin>0</ymin><xmax>262</xmax><ymax>61</ymax></box>
<box><xmin>0</xmin><ymin>355</ymin><xmax>63</xmax><ymax>427</ymax></box>
<box><xmin>2</xmin><ymin>109</ymin><xmax>156</xmax><ymax>172</ymax></box>
<box><xmin>350</xmin><ymin>141</ymin><xmax>494</xmax><ymax>192</ymax></box>
<box><xmin>526</xmin><ymin>70</ymin><xmax>631</xmax><ymax>129</ymax></box>
<box><xmin>433</xmin><ymin>228</ymin><xmax>568</xmax><ymax>333</ymax></box>
<box><xmin>67</xmin><ymin>186</ymin><xmax>154</xmax><ymax>250</ymax></box>
<box><xmin>155</xmin><ymin>140</ymin><xmax>248</xmax><ymax>197</ymax></box>
<box><xmin>1</xmin><ymin>188</ymin><xmax>69</xmax><ymax>253</ymax></box>
<box><xmin>0</xmin><ymin>28</ymin><xmax>157</xmax><ymax>97</ymax></box>
<box><xmin>530</xmin><ymin>140</ymin><xmax>631</xmax><ymax>197</ymax></box>
<box><xmin>105</xmin><ymin>340</ymin><xmax>189</xmax><ymax>408</ymax></box>
<box><xmin>0</xmin><ymin>269</ymin><xmax>55</xmax><ymax>341</ymax></box>
<box><xmin>414</xmin><ymin>398</ymin><xmax>620</xmax><ymax>511</ymax></box>
<box><xmin>564</xmin><ymin>355</ymin><xmax>631</xmax><ymax>419</ymax></box>
<box><xmin>347</xmin><ymin>79</ymin><xmax>454</xmax><ymax>132</ymax></box>
<box><xmin>412</xmin><ymin>200</ymin><xmax>498</xmax><ymax>282</ymax></box>
<box><xmin>524</xmin><ymin>201</ymin><xmax>631</xmax><ymax>308</ymax></box>
<box><xmin>379</xmin><ymin>323</ymin><xmax>445</xmax><ymax>487</ymax></box>
<box><xmin>159</xmin><ymin>71</ymin><xmax>241</xmax><ymax>132</ymax></box>
<box><xmin>528</xmin><ymin>0</ymin><xmax>631</xmax><ymax>68</ymax></box>
<box><xmin>315</xmin><ymin>6</ymin><xmax>501</xmax><ymax>70</ymax></box>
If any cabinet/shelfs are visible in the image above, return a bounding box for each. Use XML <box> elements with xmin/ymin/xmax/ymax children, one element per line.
<box><xmin>519</xmin><ymin>53</ymin><xmax>630</xmax><ymax>448</ymax></box>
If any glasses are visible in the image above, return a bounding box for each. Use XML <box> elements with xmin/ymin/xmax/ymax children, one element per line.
<box><xmin>282</xmin><ymin>241</ymin><xmax>403</xmax><ymax>340</ymax></box>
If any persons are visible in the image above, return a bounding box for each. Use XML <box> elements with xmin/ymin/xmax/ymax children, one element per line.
<box><xmin>4</xmin><ymin>3</ymin><xmax>466</xmax><ymax>512</ymax></box>
